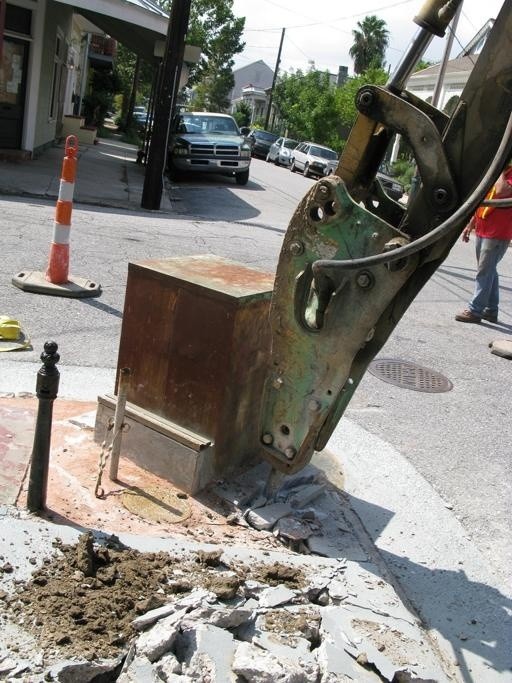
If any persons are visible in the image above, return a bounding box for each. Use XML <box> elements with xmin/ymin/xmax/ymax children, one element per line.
<box><xmin>454</xmin><ymin>163</ymin><xmax>512</xmax><ymax>323</ymax></box>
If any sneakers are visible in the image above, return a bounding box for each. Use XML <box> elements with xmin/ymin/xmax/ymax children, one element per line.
<box><xmin>455</xmin><ymin>309</ymin><xmax>499</xmax><ymax>324</ymax></box>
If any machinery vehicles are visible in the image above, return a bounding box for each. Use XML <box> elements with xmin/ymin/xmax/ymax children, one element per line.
<box><xmin>256</xmin><ymin>0</ymin><xmax>512</xmax><ymax>500</ymax></box>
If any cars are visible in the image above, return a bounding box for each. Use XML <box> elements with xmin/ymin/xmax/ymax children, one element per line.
<box><xmin>171</xmin><ymin>112</ymin><xmax>251</xmax><ymax>185</ymax></box>
<box><xmin>132</xmin><ymin>106</ymin><xmax>151</xmax><ymax>125</ymax></box>
<box><xmin>239</xmin><ymin>126</ymin><xmax>405</xmax><ymax>202</ymax></box>
